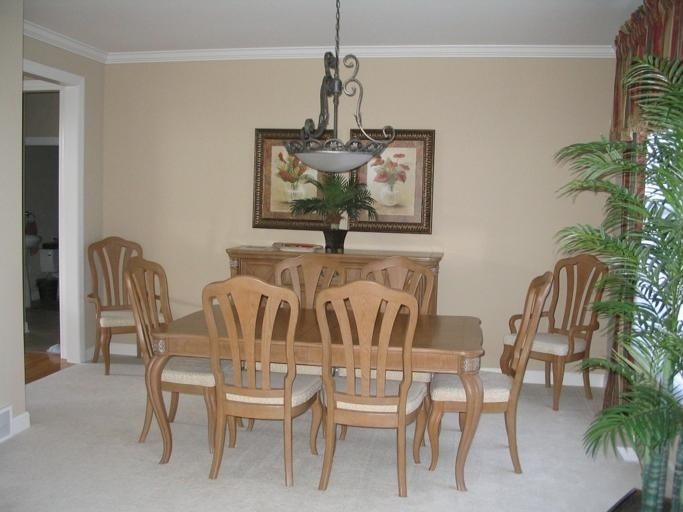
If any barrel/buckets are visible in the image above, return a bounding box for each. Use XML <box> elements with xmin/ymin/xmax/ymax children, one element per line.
<box><xmin>35</xmin><ymin>277</ymin><xmax>57</xmax><ymax>302</ymax></box>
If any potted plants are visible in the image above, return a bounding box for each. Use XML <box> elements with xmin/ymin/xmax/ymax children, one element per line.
<box><xmin>554</xmin><ymin>55</ymin><xmax>683</xmax><ymax>512</ymax></box>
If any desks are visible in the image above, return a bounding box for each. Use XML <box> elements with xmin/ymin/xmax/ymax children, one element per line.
<box><xmin>226</xmin><ymin>246</ymin><xmax>443</xmax><ymax>314</ymax></box>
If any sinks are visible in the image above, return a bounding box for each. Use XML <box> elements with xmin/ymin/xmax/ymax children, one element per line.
<box><xmin>26</xmin><ymin>234</ymin><xmax>41</xmax><ymax>248</ymax></box>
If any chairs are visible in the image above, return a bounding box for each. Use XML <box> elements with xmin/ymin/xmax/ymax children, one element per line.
<box><xmin>241</xmin><ymin>254</ymin><xmax>351</xmax><ymax>376</ymax></box>
<box><xmin>315</xmin><ymin>280</ymin><xmax>431</xmax><ymax>498</ymax></box>
<box><xmin>501</xmin><ymin>255</ymin><xmax>608</xmax><ymax>407</ymax></box>
<box><xmin>125</xmin><ymin>256</ymin><xmax>218</xmax><ymax>455</ymax></box>
<box><xmin>427</xmin><ymin>272</ymin><xmax>554</xmax><ymax>473</ymax></box>
<box><xmin>87</xmin><ymin>236</ymin><xmax>162</xmax><ymax>374</ymax></box>
<box><xmin>343</xmin><ymin>256</ymin><xmax>434</xmax><ymax>382</ymax></box>
<box><xmin>202</xmin><ymin>275</ymin><xmax>322</xmax><ymax>487</ymax></box>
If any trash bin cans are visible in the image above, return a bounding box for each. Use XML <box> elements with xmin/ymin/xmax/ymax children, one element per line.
<box><xmin>36</xmin><ymin>279</ymin><xmax>58</xmax><ymax>304</ymax></box>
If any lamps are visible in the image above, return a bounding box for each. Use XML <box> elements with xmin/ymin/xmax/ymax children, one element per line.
<box><xmin>281</xmin><ymin>1</ymin><xmax>396</xmax><ymax>172</ymax></box>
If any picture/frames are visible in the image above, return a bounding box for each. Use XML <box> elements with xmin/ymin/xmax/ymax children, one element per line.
<box><xmin>348</xmin><ymin>128</ymin><xmax>434</xmax><ymax>235</ymax></box>
<box><xmin>253</xmin><ymin>129</ymin><xmax>337</xmax><ymax>232</ymax></box>
